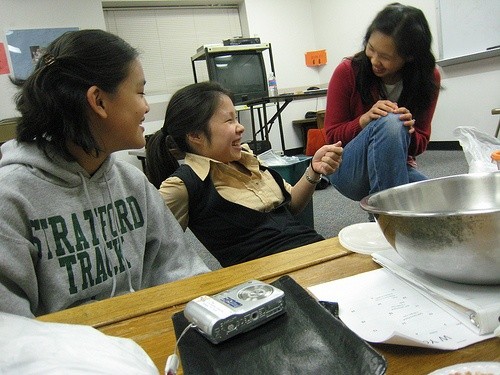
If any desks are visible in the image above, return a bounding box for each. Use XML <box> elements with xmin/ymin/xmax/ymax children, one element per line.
<box><xmin>247</xmin><ymin>92</ymin><xmax>325</xmax><ymax>155</ymax></box>
<box><xmin>29</xmin><ymin>237</ymin><xmax>500</xmax><ymax>375</ymax></box>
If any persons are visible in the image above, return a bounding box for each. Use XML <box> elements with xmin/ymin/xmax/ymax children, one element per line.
<box><xmin>0</xmin><ymin>30</ymin><xmax>214</xmax><ymax>319</ymax></box>
<box><xmin>324</xmin><ymin>1</ymin><xmax>441</xmax><ymax>224</ymax></box>
<box><xmin>146</xmin><ymin>80</ymin><xmax>345</xmax><ymax>269</ymax></box>
<box><xmin>0</xmin><ymin>311</ymin><xmax>160</xmax><ymax>375</ymax></box>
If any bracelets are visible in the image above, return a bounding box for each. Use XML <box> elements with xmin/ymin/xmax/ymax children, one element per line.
<box><xmin>310</xmin><ymin>156</ymin><xmax>322</xmax><ymax>175</ymax></box>
<box><xmin>304</xmin><ymin>164</ymin><xmax>323</xmax><ymax>184</ymax></box>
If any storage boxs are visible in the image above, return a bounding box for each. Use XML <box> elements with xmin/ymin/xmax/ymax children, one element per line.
<box><xmin>267</xmin><ymin>156</ymin><xmax>314</xmax><ymax>187</ymax></box>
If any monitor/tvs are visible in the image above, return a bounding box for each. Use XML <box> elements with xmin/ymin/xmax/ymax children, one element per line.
<box><xmin>206</xmin><ymin>50</ymin><xmax>269</xmax><ymax>104</ymax></box>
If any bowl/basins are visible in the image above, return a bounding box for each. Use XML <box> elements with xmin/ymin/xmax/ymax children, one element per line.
<box><xmin>359</xmin><ymin>173</ymin><xmax>500</xmax><ymax>285</ymax></box>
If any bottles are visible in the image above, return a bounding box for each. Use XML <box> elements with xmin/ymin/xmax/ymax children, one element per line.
<box><xmin>268</xmin><ymin>73</ymin><xmax>278</xmax><ymax>96</ymax></box>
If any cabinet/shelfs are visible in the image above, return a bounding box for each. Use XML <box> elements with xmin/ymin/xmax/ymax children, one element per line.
<box><xmin>190</xmin><ymin>43</ymin><xmax>287</xmax><ymax>157</ymax></box>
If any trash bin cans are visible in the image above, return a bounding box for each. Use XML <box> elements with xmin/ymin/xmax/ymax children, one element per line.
<box><xmin>259</xmin><ymin>155</ymin><xmax>315</xmax><ymax>229</ymax></box>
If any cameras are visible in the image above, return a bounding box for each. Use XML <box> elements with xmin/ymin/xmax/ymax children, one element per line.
<box><xmin>185</xmin><ymin>280</ymin><xmax>287</xmax><ymax>345</ymax></box>
